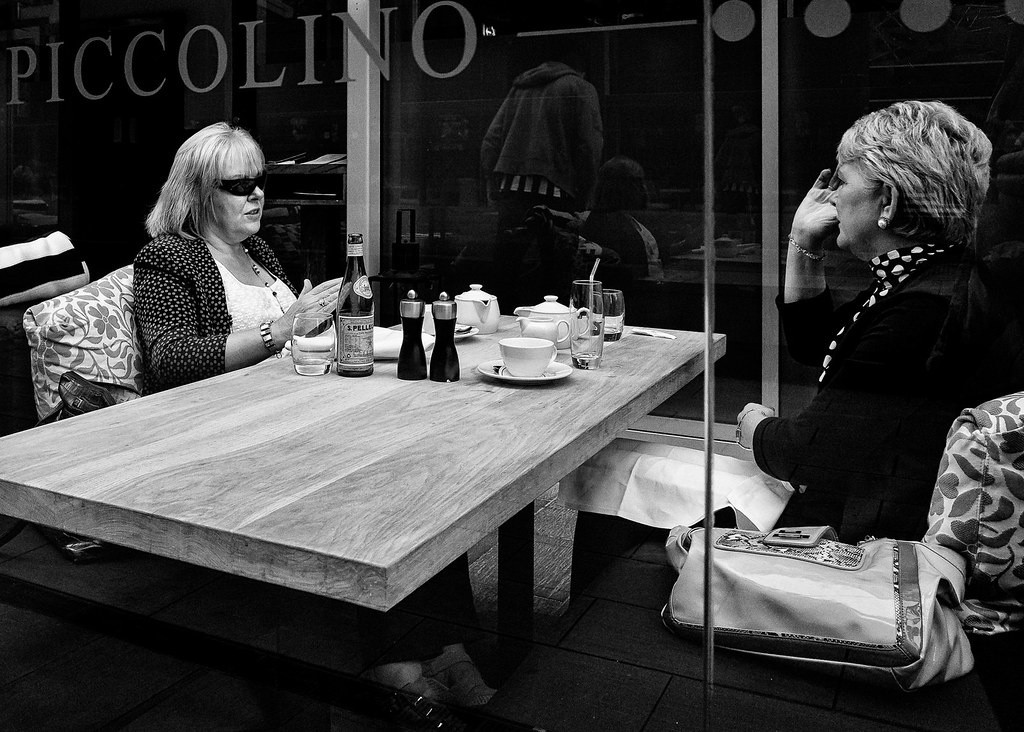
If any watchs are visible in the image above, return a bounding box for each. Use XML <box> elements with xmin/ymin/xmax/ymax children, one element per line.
<box><xmin>735</xmin><ymin>411</ymin><xmax>767</xmax><ymax>452</ymax></box>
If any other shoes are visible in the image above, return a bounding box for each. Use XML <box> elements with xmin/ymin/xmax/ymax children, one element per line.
<box><xmin>398</xmin><ymin>652</ymin><xmax>499</xmax><ymax>710</ymax></box>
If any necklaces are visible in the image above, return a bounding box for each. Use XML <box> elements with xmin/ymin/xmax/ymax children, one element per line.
<box><xmin>229</xmin><ymin>244</ymin><xmax>285</xmax><ymax>333</ymax></box>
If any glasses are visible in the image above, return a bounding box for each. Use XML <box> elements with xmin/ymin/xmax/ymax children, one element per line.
<box><xmin>214</xmin><ymin>174</ymin><xmax>267</xmax><ymax>196</ymax></box>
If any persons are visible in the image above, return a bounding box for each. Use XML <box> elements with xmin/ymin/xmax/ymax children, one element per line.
<box><xmin>660</xmin><ymin>100</ymin><xmax>1024</xmax><ymax>649</ymax></box>
<box><xmin>135</xmin><ymin>122</ymin><xmax>534</xmax><ymax>732</ymax></box>
<box><xmin>476</xmin><ymin>50</ymin><xmax>668</xmax><ymax>328</ymax></box>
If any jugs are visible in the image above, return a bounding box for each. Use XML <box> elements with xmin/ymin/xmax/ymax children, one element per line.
<box><xmin>514</xmin><ymin>295</ymin><xmax>590</xmax><ymax>350</ymax></box>
<box><xmin>454</xmin><ymin>284</ymin><xmax>500</xmax><ymax>334</ymax></box>
<box><xmin>516</xmin><ymin>315</ymin><xmax>571</xmax><ymax>347</ymax></box>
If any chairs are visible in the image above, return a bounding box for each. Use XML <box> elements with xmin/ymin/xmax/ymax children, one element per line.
<box><xmin>658</xmin><ymin>391</ymin><xmax>1024</xmax><ymax>691</ymax></box>
<box><xmin>23</xmin><ymin>261</ymin><xmax>146</xmax><ymax>429</ymax></box>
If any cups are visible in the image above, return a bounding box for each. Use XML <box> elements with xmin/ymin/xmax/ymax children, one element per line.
<box><xmin>568</xmin><ymin>279</ymin><xmax>605</xmax><ymax>370</ymax></box>
<box><xmin>498</xmin><ymin>338</ymin><xmax>558</xmax><ymax>378</ymax></box>
<box><xmin>291</xmin><ymin>312</ymin><xmax>334</xmax><ymax>376</ymax></box>
<box><xmin>601</xmin><ymin>288</ymin><xmax>625</xmax><ymax>342</ymax></box>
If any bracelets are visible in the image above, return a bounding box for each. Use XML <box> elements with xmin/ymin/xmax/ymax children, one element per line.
<box><xmin>788</xmin><ymin>234</ymin><xmax>827</xmax><ymax>263</ymax></box>
<box><xmin>259</xmin><ymin>320</ymin><xmax>279</xmax><ymax>354</ymax></box>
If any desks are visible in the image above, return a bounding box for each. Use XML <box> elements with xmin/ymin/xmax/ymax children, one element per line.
<box><xmin>0</xmin><ymin>307</ymin><xmax>725</xmax><ymax>732</ymax></box>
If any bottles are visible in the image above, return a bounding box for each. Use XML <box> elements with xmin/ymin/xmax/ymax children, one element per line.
<box><xmin>335</xmin><ymin>233</ymin><xmax>375</xmax><ymax>377</ymax></box>
<box><xmin>396</xmin><ymin>290</ymin><xmax>427</xmax><ymax>380</ymax></box>
<box><xmin>429</xmin><ymin>291</ymin><xmax>460</xmax><ymax>382</ymax></box>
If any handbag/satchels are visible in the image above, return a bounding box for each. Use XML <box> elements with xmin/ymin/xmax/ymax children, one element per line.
<box><xmin>662</xmin><ymin>521</ymin><xmax>976</xmax><ymax>687</ymax></box>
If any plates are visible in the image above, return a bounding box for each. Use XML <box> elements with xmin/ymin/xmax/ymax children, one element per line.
<box><xmin>455</xmin><ymin>324</ymin><xmax>479</xmax><ymax>342</ymax></box>
<box><xmin>477</xmin><ymin>359</ymin><xmax>572</xmax><ymax>381</ymax></box>
